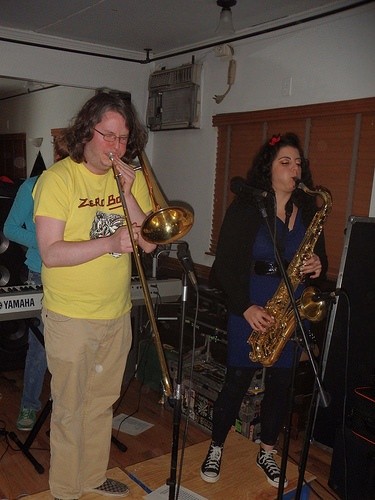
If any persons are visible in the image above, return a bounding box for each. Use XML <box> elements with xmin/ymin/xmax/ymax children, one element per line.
<box><xmin>198</xmin><ymin>131</ymin><xmax>331</xmax><ymax>490</ymax></box>
<box><xmin>34</xmin><ymin>89</ymin><xmax>159</xmax><ymax>500</ymax></box>
<box><xmin>2</xmin><ymin>128</ymin><xmax>86</xmax><ymax>431</ymax></box>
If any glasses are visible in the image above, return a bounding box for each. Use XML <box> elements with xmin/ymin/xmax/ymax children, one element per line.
<box><xmin>94</xmin><ymin>128</ymin><xmax>131</xmax><ymax>144</ymax></box>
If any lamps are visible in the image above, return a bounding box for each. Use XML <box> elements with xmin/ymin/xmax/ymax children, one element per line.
<box><xmin>215</xmin><ymin>0</ymin><xmax>237</xmax><ymax>35</ymax></box>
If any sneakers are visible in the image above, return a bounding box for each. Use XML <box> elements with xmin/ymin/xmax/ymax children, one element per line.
<box><xmin>201</xmin><ymin>441</ymin><xmax>223</xmax><ymax>483</ymax></box>
<box><xmin>256</xmin><ymin>446</ymin><xmax>288</xmax><ymax>488</ymax></box>
<box><xmin>17</xmin><ymin>407</ymin><xmax>41</xmax><ymax>430</ymax></box>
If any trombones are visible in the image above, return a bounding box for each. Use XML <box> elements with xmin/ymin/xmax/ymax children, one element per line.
<box><xmin>108</xmin><ymin>148</ymin><xmax>194</xmax><ymax>396</ymax></box>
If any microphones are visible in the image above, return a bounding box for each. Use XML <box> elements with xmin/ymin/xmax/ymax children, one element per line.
<box><xmin>178</xmin><ymin>251</ymin><xmax>200</xmax><ymax>290</ymax></box>
<box><xmin>229</xmin><ymin>180</ymin><xmax>269</xmax><ymax>199</ymax></box>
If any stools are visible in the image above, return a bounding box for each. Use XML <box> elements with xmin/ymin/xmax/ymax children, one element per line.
<box><xmin>141</xmin><ymin>425</ymin><xmax>317</xmax><ymax>500</ymax></box>
<box><xmin>13</xmin><ymin>468</ymin><xmax>157</xmax><ymax>500</ymax></box>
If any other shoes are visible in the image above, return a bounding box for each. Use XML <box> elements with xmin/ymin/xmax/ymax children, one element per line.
<box><xmin>92</xmin><ymin>478</ymin><xmax>129</xmax><ymax>496</ymax></box>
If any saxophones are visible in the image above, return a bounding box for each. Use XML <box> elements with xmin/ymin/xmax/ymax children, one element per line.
<box><xmin>246</xmin><ymin>177</ymin><xmax>326</xmax><ymax>367</ymax></box>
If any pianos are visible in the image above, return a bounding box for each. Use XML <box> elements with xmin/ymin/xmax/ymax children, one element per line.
<box><xmin>0</xmin><ymin>275</ymin><xmax>184</xmax><ymax>473</ymax></box>
